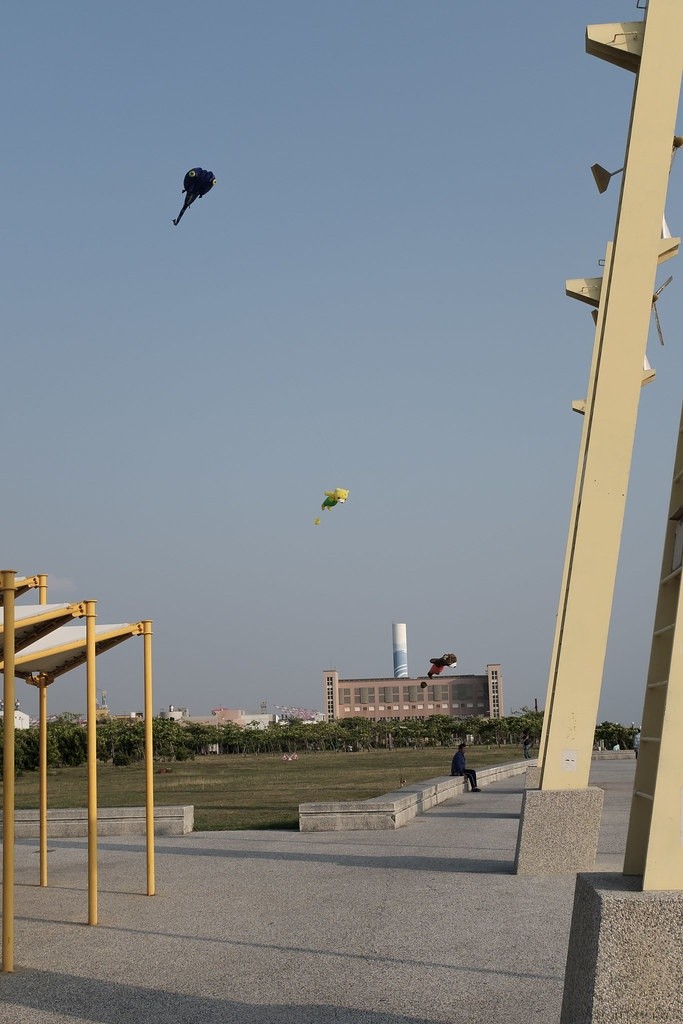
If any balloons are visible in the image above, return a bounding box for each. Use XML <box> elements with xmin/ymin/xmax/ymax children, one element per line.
<box><xmin>421</xmin><ymin>654</ymin><xmax>458</xmax><ymax>689</ymax></box>
<box><xmin>314</xmin><ymin>488</ymin><xmax>349</xmax><ymax>526</ymax></box>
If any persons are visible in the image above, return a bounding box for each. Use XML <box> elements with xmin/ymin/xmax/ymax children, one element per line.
<box><xmin>522</xmin><ymin>735</ymin><xmax>531</xmax><ymax>759</ymax></box>
<box><xmin>451</xmin><ymin>744</ymin><xmax>481</xmax><ymax>792</ymax></box>
<box><xmin>633</xmin><ymin>729</ymin><xmax>641</xmax><ymax>758</ymax></box>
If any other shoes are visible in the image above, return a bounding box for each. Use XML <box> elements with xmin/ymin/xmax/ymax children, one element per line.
<box><xmin>472</xmin><ymin>788</ymin><xmax>481</xmax><ymax>792</ymax></box>
